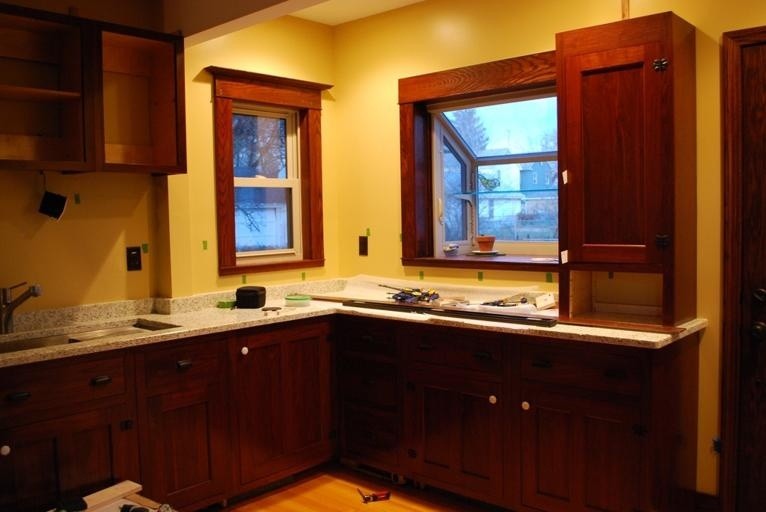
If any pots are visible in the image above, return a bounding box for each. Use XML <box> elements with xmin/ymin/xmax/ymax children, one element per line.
<box><xmin>38</xmin><ymin>170</ymin><xmax>68</xmax><ymax>222</ymax></box>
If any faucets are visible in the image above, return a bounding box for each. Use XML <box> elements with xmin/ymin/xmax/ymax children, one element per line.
<box><xmin>0</xmin><ymin>281</ymin><xmax>42</xmax><ymax>334</ymax></box>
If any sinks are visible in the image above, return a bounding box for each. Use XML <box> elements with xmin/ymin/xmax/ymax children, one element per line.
<box><xmin>0</xmin><ymin>318</ymin><xmax>184</xmax><ymax>352</ymax></box>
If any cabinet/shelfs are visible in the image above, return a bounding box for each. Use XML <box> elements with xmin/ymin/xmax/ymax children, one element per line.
<box><xmin>0</xmin><ymin>3</ymin><xmax>187</xmax><ymax>175</ymax></box>
<box><xmin>556</xmin><ymin>11</ymin><xmax>696</xmax><ymax>326</ymax></box>
<box><xmin>136</xmin><ymin>330</ymin><xmax>233</xmax><ymax>512</ymax></box>
<box><xmin>0</xmin><ymin>345</ymin><xmax>141</xmax><ymax>512</ymax></box>
<box><xmin>227</xmin><ymin>314</ymin><xmax>335</xmax><ymax>497</ymax></box>
<box><xmin>336</xmin><ymin>314</ymin><xmax>699</xmax><ymax>512</ymax></box>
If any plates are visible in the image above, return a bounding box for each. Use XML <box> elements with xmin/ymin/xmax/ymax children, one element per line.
<box><xmin>473</xmin><ymin>250</ymin><xmax>499</xmax><ymax>255</ymax></box>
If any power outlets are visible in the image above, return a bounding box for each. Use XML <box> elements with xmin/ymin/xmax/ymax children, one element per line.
<box><xmin>127</xmin><ymin>247</ymin><xmax>141</xmax><ymax>270</ymax></box>
